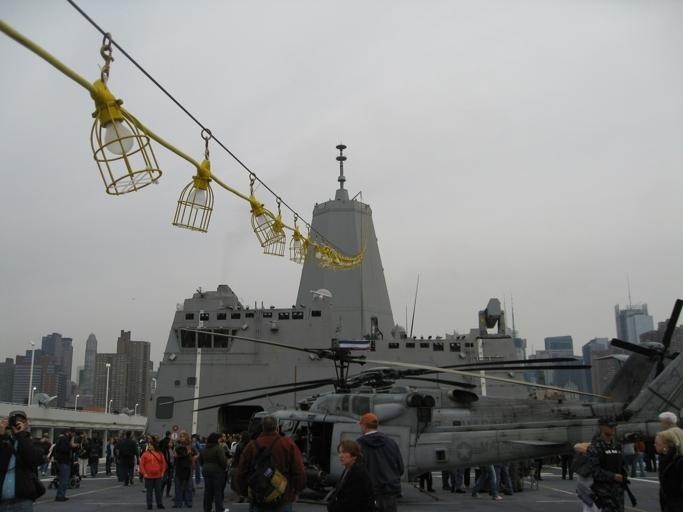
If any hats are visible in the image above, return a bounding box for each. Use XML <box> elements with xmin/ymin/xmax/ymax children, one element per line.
<box><xmin>7</xmin><ymin>410</ymin><xmax>27</xmax><ymax>422</ymax></box>
<box><xmin>599</xmin><ymin>414</ymin><xmax>618</xmax><ymax>427</ymax></box>
<box><xmin>356</xmin><ymin>413</ymin><xmax>379</xmax><ymax>427</ymax></box>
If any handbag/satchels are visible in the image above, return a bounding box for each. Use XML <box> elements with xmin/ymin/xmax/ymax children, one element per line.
<box><xmin>23</xmin><ymin>472</ymin><xmax>45</xmax><ymax>501</ymax></box>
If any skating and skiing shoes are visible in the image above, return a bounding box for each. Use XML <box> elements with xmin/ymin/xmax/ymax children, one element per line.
<box><xmin>419</xmin><ymin>477</ymin><xmax>576</xmax><ymax>501</ymax></box>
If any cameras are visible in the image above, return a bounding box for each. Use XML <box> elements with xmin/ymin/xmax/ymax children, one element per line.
<box><xmin>8</xmin><ymin>414</ymin><xmax>17</xmax><ymax>426</ymax></box>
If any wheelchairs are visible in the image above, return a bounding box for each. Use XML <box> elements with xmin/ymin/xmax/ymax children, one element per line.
<box><xmin>51</xmin><ymin>461</ymin><xmax>83</xmax><ymax>491</ymax></box>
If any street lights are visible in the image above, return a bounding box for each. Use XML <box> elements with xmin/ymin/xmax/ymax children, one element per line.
<box><xmin>26</xmin><ymin>341</ymin><xmax>37</xmax><ymax>407</ymax></box>
<box><xmin>150</xmin><ymin>378</ymin><xmax>157</xmax><ymax>390</ymax></box>
<box><xmin>105</xmin><ymin>362</ymin><xmax>111</xmax><ymax>413</ymax></box>
<box><xmin>74</xmin><ymin>394</ymin><xmax>81</xmax><ymax>411</ymax></box>
<box><xmin>31</xmin><ymin>385</ymin><xmax>37</xmax><ymax>406</ymax></box>
<box><xmin>108</xmin><ymin>399</ymin><xmax>113</xmax><ymax>413</ymax></box>
<box><xmin>134</xmin><ymin>404</ymin><xmax>139</xmax><ymax>416</ymax></box>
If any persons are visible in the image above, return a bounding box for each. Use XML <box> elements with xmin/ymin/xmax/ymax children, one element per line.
<box><xmin>1</xmin><ymin>411</ymin><xmax>404</xmax><ymax>511</ymax></box>
<box><xmin>419</xmin><ymin>411</ymin><xmax>683</xmax><ymax>512</ymax></box>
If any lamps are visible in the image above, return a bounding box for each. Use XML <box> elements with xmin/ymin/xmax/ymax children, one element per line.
<box><xmin>263</xmin><ymin>215</ymin><xmax>286</xmax><ymax>257</ymax></box>
<box><xmin>89</xmin><ymin>78</ymin><xmax>164</xmax><ymax>196</ymax></box>
<box><xmin>248</xmin><ymin>194</ymin><xmax>286</xmax><ymax>247</ymax></box>
<box><xmin>302</xmin><ymin>238</ymin><xmax>365</xmax><ymax>273</ymax></box>
<box><xmin>171</xmin><ymin>157</ymin><xmax>215</xmax><ymax>233</ymax></box>
<box><xmin>288</xmin><ymin>226</ymin><xmax>307</xmax><ymax>264</ymax></box>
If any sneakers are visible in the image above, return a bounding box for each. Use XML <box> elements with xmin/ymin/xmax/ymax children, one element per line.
<box><xmin>55</xmin><ymin>496</ymin><xmax>69</xmax><ymax>502</ymax></box>
<box><xmin>141</xmin><ymin>485</ymin><xmax>231</xmax><ymax>512</ymax></box>
<box><xmin>90</xmin><ymin>471</ymin><xmax>135</xmax><ymax>486</ymax></box>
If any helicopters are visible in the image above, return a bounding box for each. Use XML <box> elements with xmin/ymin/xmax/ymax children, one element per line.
<box><xmin>156</xmin><ymin>297</ymin><xmax>683</xmax><ymax>503</ymax></box>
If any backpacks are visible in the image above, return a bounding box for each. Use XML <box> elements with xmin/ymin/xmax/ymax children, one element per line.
<box><xmin>244</xmin><ymin>436</ymin><xmax>288</xmax><ymax>504</ymax></box>
